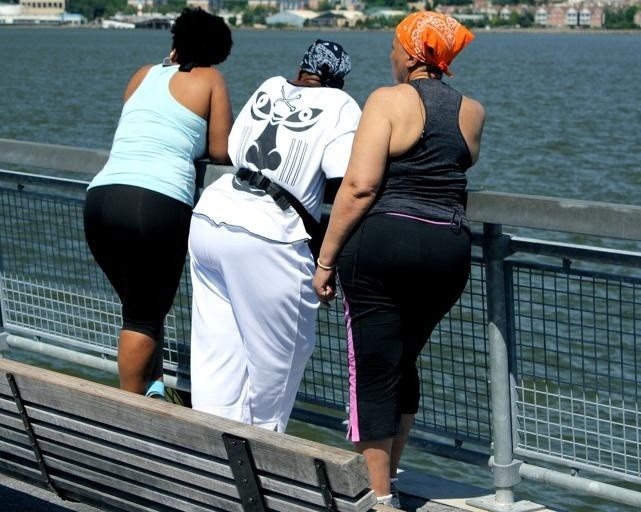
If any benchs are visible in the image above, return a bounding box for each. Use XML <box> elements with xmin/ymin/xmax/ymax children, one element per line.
<box><xmin>0</xmin><ymin>356</ymin><xmax>382</xmax><ymax>512</ymax></box>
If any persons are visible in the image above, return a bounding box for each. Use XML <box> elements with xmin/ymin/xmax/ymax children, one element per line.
<box><xmin>82</xmin><ymin>8</ymin><xmax>236</xmax><ymax>401</ymax></box>
<box><xmin>187</xmin><ymin>39</ymin><xmax>363</xmax><ymax>434</ymax></box>
<box><xmin>311</xmin><ymin>8</ymin><xmax>485</xmax><ymax>512</ymax></box>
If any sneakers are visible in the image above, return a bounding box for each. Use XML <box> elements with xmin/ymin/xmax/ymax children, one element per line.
<box><xmin>143</xmin><ymin>381</ymin><xmax>166</xmax><ymax>400</ymax></box>
<box><xmin>376</xmin><ymin>480</ymin><xmax>401</xmax><ymax>510</ymax></box>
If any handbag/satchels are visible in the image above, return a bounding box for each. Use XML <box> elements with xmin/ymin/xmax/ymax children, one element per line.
<box><xmin>308</xmin><ymin>214</ymin><xmax>336</xmax><ymax>293</ymax></box>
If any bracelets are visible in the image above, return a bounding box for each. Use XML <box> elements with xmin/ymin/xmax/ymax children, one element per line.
<box><xmin>314</xmin><ymin>257</ymin><xmax>336</xmax><ymax>270</ymax></box>
